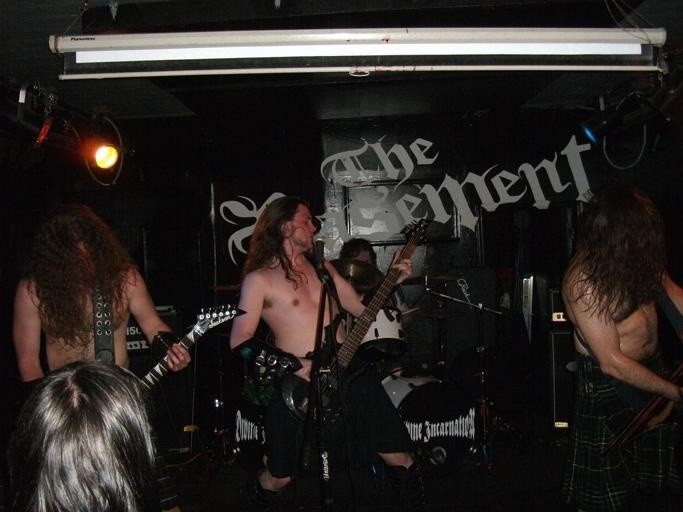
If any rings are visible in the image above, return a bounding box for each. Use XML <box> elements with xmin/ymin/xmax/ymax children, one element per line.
<box><xmin>407</xmin><ymin>263</ymin><xmax>411</xmax><ymax>266</ymax></box>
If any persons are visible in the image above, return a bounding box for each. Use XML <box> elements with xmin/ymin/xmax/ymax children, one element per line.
<box><xmin>12</xmin><ymin>359</ymin><xmax>156</xmax><ymax>512</ymax></box>
<box><xmin>560</xmin><ymin>182</ymin><xmax>683</xmax><ymax>511</ymax></box>
<box><xmin>228</xmin><ymin>195</ymin><xmax>427</xmax><ymax>511</ymax></box>
<box><xmin>12</xmin><ymin>202</ymin><xmax>193</xmax><ymax>511</ymax></box>
<box><xmin>332</xmin><ymin>239</ymin><xmax>412</xmax><ymax>345</ymax></box>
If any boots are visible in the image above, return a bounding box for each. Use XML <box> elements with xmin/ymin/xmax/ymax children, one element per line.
<box><xmin>387</xmin><ymin>461</ymin><xmax>427</xmax><ymax>511</ymax></box>
<box><xmin>236</xmin><ymin>476</ymin><xmax>277</xmax><ymax>511</ymax></box>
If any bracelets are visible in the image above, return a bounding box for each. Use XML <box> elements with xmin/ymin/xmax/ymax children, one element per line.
<box><xmin>150</xmin><ymin>331</ymin><xmax>178</xmax><ymax>356</ymax></box>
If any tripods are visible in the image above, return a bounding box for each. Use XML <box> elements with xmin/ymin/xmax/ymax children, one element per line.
<box><xmin>428</xmin><ymin>290</ymin><xmax>523</xmax><ymax>472</ymax></box>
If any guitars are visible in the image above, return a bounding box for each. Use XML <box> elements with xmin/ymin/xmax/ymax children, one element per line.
<box><xmin>282</xmin><ymin>218</ymin><xmax>434</xmax><ymax>434</ymax></box>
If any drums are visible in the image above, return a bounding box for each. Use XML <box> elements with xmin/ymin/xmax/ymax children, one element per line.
<box><xmin>374</xmin><ymin>364</ymin><xmax>481</xmax><ymax>481</ymax></box>
<box><xmin>349</xmin><ymin>307</ymin><xmax>415</xmax><ymax>363</ymax></box>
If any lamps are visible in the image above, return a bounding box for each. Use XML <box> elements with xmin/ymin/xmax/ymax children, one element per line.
<box><xmin>66</xmin><ymin>134</ymin><xmax>126</xmax><ymax>178</ymax></box>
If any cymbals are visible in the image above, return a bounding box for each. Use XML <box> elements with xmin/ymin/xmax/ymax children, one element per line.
<box><xmin>328</xmin><ymin>257</ymin><xmax>385</xmax><ymax>292</ymax></box>
<box><xmin>401</xmin><ymin>274</ymin><xmax>456</xmax><ymax>286</ymax></box>
<box><xmin>414</xmin><ymin>306</ymin><xmax>470</xmax><ymax>319</ymax></box>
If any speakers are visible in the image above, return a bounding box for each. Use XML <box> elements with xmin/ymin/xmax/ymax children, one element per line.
<box><xmin>548</xmin><ymin>325</ymin><xmax>575</xmax><ymax>430</ymax></box>
<box><xmin>547</xmin><ymin>287</ymin><xmax>570</xmax><ymax>324</ymax></box>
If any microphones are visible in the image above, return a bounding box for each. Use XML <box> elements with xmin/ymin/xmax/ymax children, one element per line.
<box><xmin>423</xmin><ymin>265</ymin><xmax>432</xmax><ymax>294</ymax></box>
<box><xmin>311</xmin><ymin>240</ymin><xmax>325</xmax><ymax>282</ymax></box>
